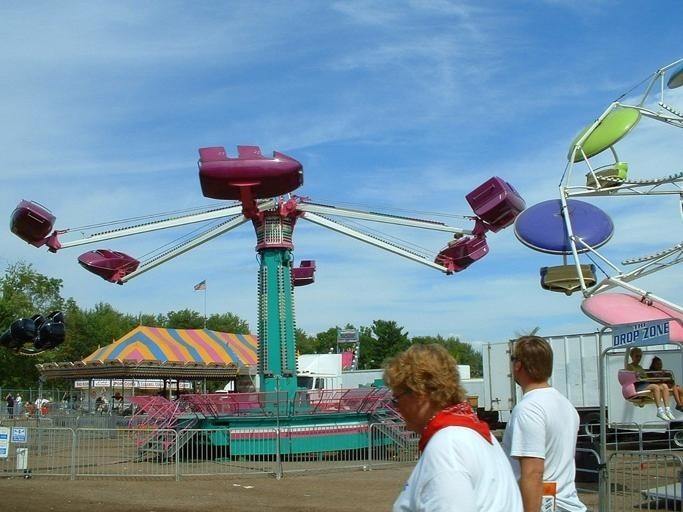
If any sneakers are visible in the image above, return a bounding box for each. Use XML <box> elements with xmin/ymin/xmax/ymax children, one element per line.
<box><xmin>656</xmin><ymin>404</ymin><xmax>683</xmax><ymax>421</ymax></box>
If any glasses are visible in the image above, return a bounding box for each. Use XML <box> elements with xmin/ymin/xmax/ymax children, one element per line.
<box><xmin>509</xmin><ymin>355</ymin><xmax>519</xmax><ymax>360</ymax></box>
<box><xmin>390</xmin><ymin>388</ymin><xmax>413</xmax><ymax>406</ymax></box>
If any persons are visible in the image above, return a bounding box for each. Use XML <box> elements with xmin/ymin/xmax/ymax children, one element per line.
<box><xmin>501</xmin><ymin>335</ymin><xmax>587</xmax><ymax>511</ymax></box>
<box><xmin>448</xmin><ymin>233</ymin><xmax>463</xmax><ymax>246</ymax></box>
<box><xmin>648</xmin><ymin>355</ymin><xmax>682</xmax><ymax>413</ymax></box>
<box><xmin>1</xmin><ymin>384</ymin><xmax>202</xmax><ymax>419</ymax></box>
<box><xmin>626</xmin><ymin>346</ymin><xmax>676</xmax><ymax>421</ymax></box>
<box><xmin>380</xmin><ymin>342</ymin><xmax>524</xmax><ymax>512</ymax></box>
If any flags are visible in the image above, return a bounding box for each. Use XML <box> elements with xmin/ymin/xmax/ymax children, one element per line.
<box><xmin>194</xmin><ymin>279</ymin><xmax>207</xmax><ymax>290</ymax></box>
